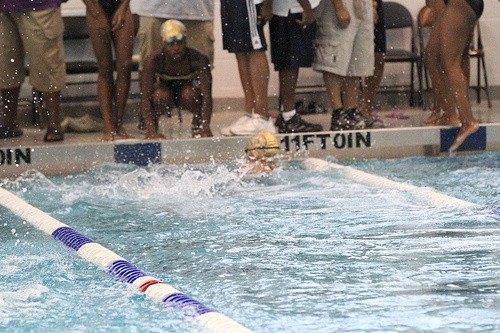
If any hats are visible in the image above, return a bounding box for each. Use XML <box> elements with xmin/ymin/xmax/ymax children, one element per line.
<box><xmin>160</xmin><ymin>20</ymin><xmax>187</xmax><ymax>42</ymax></box>
<box><xmin>247</xmin><ymin>131</ymin><xmax>278</xmax><ymax>158</ymax></box>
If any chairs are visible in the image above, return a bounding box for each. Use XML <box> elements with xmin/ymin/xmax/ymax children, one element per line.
<box><xmin>23</xmin><ymin>13</ymin><xmax>147</xmax><ymax>131</ymax></box>
<box><xmin>358</xmin><ymin>2</ymin><xmax>493</xmax><ymax>109</ymax></box>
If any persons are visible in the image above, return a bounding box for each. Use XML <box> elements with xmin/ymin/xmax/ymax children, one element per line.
<box><xmin>312</xmin><ymin>0</ymin><xmax>387</xmax><ymax>132</ymax></box>
<box><xmin>0</xmin><ymin>0</ymin><xmax>68</xmax><ymax>142</ymax></box>
<box><xmin>220</xmin><ymin>0</ymin><xmax>275</xmax><ymax>136</ymax></box>
<box><xmin>81</xmin><ymin>0</ymin><xmax>134</xmax><ymax>143</ymax></box>
<box><xmin>238</xmin><ymin>131</ymin><xmax>280</xmax><ymax>174</ymax></box>
<box><xmin>129</xmin><ymin>0</ymin><xmax>215</xmax><ymax>130</ymax></box>
<box><xmin>140</xmin><ymin>19</ymin><xmax>214</xmax><ymax>138</ymax></box>
<box><xmin>268</xmin><ymin>0</ymin><xmax>322</xmax><ymax>134</ymax></box>
<box><xmin>420</xmin><ymin>0</ymin><xmax>484</xmax><ymax>153</ymax></box>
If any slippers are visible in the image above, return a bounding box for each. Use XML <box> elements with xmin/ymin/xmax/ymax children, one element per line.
<box><xmin>0</xmin><ymin>128</ymin><xmax>23</xmax><ymax>138</ymax></box>
<box><xmin>44</xmin><ymin>128</ymin><xmax>63</xmax><ymax>142</ymax></box>
<box><xmin>297</xmin><ymin>99</ymin><xmax>327</xmax><ymax>115</ymax></box>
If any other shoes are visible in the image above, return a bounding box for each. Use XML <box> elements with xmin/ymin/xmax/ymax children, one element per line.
<box><xmin>221</xmin><ymin>115</ymin><xmax>250</xmax><ymax>135</ymax></box>
<box><xmin>276</xmin><ymin>113</ymin><xmax>323</xmax><ymax>133</ymax></box>
<box><xmin>230</xmin><ymin>107</ymin><xmax>275</xmax><ymax>136</ymax></box>
<box><xmin>330</xmin><ymin>111</ymin><xmax>356</xmax><ymax>130</ymax></box>
<box><xmin>61</xmin><ymin>114</ymin><xmax>100</xmax><ymax>132</ymax></box>
<box><xmin>348</xmin><ymin>107</ymin><xmax>366</xmax><ymax>127</ymax></box>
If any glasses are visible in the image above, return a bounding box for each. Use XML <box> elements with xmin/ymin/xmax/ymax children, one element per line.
<box><xmin>166</xmin><ymin>34</ymin><xmax>185</xmax><ymax>45</ymax></box>
